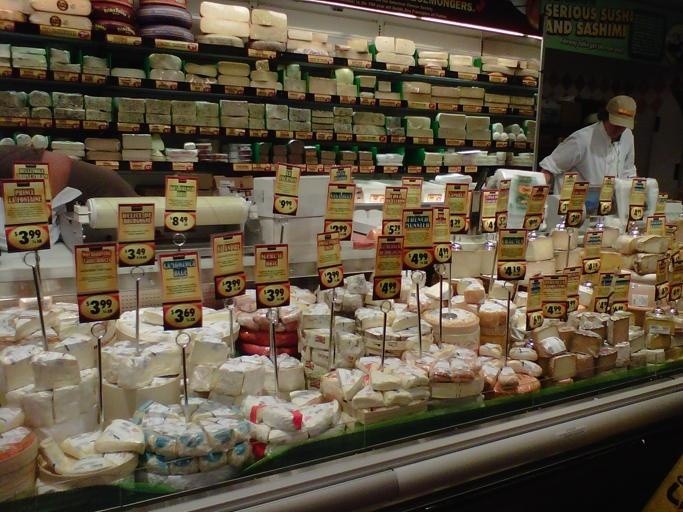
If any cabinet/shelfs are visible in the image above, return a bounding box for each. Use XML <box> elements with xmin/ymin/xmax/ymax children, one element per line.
<box><xmin>0</xmin><ymin>279</ymin><xmax>683</xmax><ymax>512</ymax></box>
<box><xmin>1</xmin><ymin>1</ymin><xmax>547</xmax><ymax>306</ymax></box>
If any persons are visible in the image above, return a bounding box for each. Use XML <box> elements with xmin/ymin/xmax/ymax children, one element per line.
<box><xmin>538</xmin><ymin>95</ymin><xmax>638</xmax><ymax>195</ymax></box>
<box><xmin>0</xmin><ymin>143</ymin><xmax>140</xmax><ymax>212</ymax></box>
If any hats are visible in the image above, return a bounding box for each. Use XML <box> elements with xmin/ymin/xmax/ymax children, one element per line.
<box><xmin>606</xmin><ymin>95</ymin><xmax>636</xmax><ymax>129</ymax></box>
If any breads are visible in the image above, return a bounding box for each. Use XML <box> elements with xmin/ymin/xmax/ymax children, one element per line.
<box><xmin>665</xmin><ymin>347</ymin><xmax>682</xmax><ymax>359</ymax></box>
<box><xmin>643</xmin><ymin>316</ymin><xmax>675</xmax><ymax>335</ymax></box>
<box><xmin>465</xmin><ymin>282</ymin><xmax>486</xmax><ymax>305</ymax></box>
<box><xmin>576</xmin><ymin>353</ymin><xmax>594</xmax><ymax>378</ymax></box>
<box><xmin>478</xmin><ymin>342</ymin><xmax>503</xmax><ymax>359</ymax></box>
<box><xmin>635</xmin><ymin>234</ymin><xmax>667</xmax><ymax>253</ymax></box>
<box><xmin>492</xmin><ymin>373</ymin><xmax>540</xmax><ymax>399</ymax></box>
<box><xmin>632</xmin><ymin>253</ymin><xmax>658</xmax><ymax>275</ymax></box>
<box><xmin>566</xmin><ymin>328</ymin><xmax>601</xmax><ymax>358</ymax></box>
<box><xmin>538</xmin><ymin>337</ymin><xmax>567</xmax><ymax>358</ymax></box>
<box><xmin>533</xmin><ymin>323</ymin><xmax>560</xmax><ymax>345</ymax></box>
<box><xmin>629</xmin><ymin>354</ymin><xmax>647</xmax><ymax>369</ymax></box>
<box><xmin>646</xmin><ymin>332</ymin><xmax>672</xmax><ymax>349</ymax></box>
<box><xmin>450</xmin><ymin>296</ymin><xmax>517</xmax><ymax>329</ymax></box>
<box><xmin>596</xmin><ymin>346</ymin><xmax>617</xmax><ymax>373</ymax></box>
<box><xmin>549</xmin><ymin>353</ymin><xmax>576</xmax><ymax>380</ymax></box>
<box><xmin>607</xmin><ymin>313</ymin><xmax>630</xmax><ymax>345</ymax></box>
<box><xmin>422</xmin><ymin>307</ymin><xmax>480</xmax><ymax>334</ymax></box>
